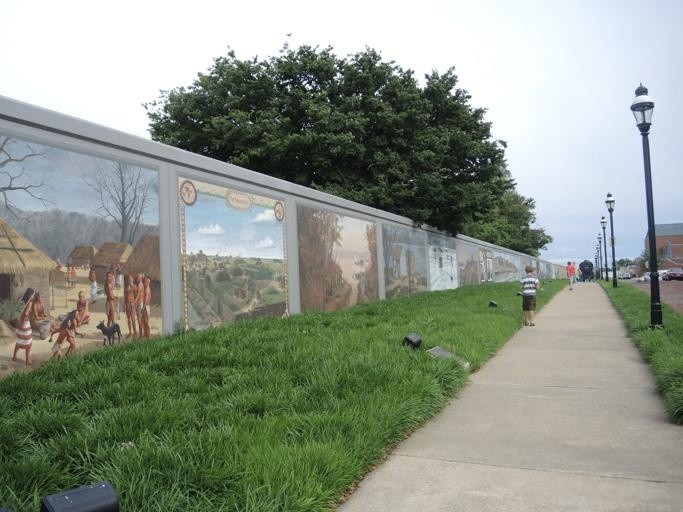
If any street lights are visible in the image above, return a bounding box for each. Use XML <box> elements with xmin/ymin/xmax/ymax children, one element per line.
<box><xmin>594</xmin><ymin>217</ymin><xmax>608</xmax><ymax>281</ymax></box>
<box><xmin>605</xmin><ymin>193</ymin><xmax>617</xmax><ymax>288</ymax></box>
<box><xmin>630</xmin><ymin>81</ymin><xmax>665</xmax><ymax>331</ymax></box>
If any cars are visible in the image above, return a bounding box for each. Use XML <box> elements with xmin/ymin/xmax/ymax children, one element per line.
<box><xmin>665</xmin><ymin>267</ymin><xmax>683</xmax><ymax>281</ymax></box>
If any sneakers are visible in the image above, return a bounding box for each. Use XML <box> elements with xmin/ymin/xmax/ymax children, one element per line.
<box><xmin>530</xmin><ymin>323</ymin><xmax>535</xmax><ymax>326</ymax></box>
<box><xmin>525</xmin><ymin>322</ymin><xmax>529</xmax><ymax>325</ymax></box>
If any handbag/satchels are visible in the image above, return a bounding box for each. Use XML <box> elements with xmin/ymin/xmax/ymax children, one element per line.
<box><xmin>9</xmin><ymin>310</ymin><xmax>24</xmax><ymax>328</ymax></box>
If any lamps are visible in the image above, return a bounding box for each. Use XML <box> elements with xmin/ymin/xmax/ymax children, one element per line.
<box><xmin>39</xmin><ymin>482</ymin><xmax>119</xmax><ymax>512</ymax></box>
<box><xmin>488</xmin><ymin>301</ymin><xmax>498</xmax><ymax>307</ymax></box>
<box><xmin>402</xmin><ymin>331</ymin><xmax>421</xmax><ymax>348</ymax></box>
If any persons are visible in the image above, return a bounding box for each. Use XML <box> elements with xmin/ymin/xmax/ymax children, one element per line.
<box><xmin>565</xmin><ymin>261</ymin><xmax>575</xmax><ymax>291</ymax></box>
<box><xmin>11</xmin><ymin>256</ymin><xmax>153</xmax><ymax>366</ymax></box>
<box><xmin>519</xmin><ymin>265</ymin><xmax>540</xmax><ymax>326</ymax></box>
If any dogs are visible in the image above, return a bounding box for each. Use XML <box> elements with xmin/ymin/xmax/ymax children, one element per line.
<box><xmin>96</xmin><ymin>320</ymin><xmax>123</xmax><ymax>347</ymax></box>
